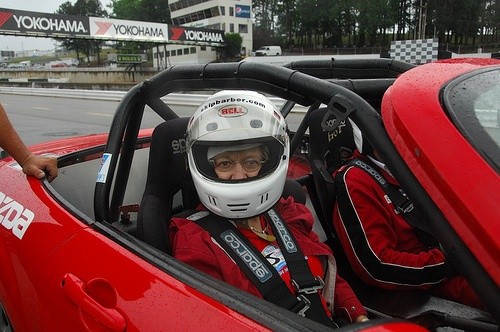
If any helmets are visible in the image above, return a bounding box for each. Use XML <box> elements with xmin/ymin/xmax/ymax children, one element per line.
<box><xmin>184</xmin><ymin>89</ymin><xmax>290</xmax><ymax>219</ymax></box>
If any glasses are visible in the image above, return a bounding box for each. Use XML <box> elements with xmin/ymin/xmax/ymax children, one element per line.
<box><xmin>214</xmin><ymin>157</ymin><xmax>268</xmax><ymax>172</ymax></box>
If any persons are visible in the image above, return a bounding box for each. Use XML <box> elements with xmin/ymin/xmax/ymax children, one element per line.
<box><xmin>333</xmin><ymin>115</ymin><xmax>483</xmax><ymax>315</ymax></box>
<box><xmin>169</xmin><ymin>89</ymin><xmax>370</xmax><ymax>329</ymax></box>
<box><xmin>0</xmin><ymin>100</ymin><xmax>60</xmax><ymax>180</ymax></box>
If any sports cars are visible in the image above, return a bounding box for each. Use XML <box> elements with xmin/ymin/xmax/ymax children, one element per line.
<box><xmin>0</xmin><ymin>58</ymin><xmax>500</xmax><ymax>332</ymax></box>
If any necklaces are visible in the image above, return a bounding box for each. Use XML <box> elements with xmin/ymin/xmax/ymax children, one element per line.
<box><xmin>235</xmin><ymin>214</ymin><xmax>268</xmax><ymax>232</ymax></box>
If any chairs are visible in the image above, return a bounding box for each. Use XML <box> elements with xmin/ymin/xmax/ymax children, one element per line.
<box><xmin>137</xmin><ymin>108</ymin><xmax>357</xmax><ymax>255</ymax></box>
